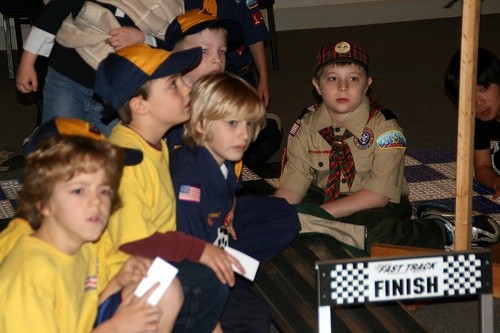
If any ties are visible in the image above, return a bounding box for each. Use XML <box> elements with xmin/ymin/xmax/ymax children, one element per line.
<box><xmin>203</xmin><ymin>0</ymin><xmax>217</xmax><ymax>16</ymax></box>
<box><xmin>318</xmin><ymin>127</ymin><xmax>356</xmax><ymax>202</ymax></box>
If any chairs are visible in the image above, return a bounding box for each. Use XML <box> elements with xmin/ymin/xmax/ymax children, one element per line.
<box><xmin>314</xmin><ymin>249</ymin><xmax>494</xmax><ymax>333</ymax></box>
<box><xmin>0</xmin><ymin>0</ymin><xmax>280</xmax><ymax>80</ymax></box>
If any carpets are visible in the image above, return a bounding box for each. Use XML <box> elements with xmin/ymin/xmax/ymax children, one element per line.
<box><xmin>249</xmin><ymin>143</ymin><xmax>500</xmax><ymax>333</ymax></box>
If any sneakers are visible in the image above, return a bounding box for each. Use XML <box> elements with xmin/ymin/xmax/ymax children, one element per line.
<box><xmin>418</xmin><ymin>209</ymin><xmax>500</xmax><ymax>247</ymax></box>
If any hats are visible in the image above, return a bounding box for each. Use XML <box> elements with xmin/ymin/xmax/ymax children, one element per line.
<box><xmin>165</xmin><ymin>13</ymin><xmax>237</xmax><ymax>50</ymax></box>
<box><xmin>95</xmin><ymin>44</ymin><xmax>203</xmax><ymax>109</ymax></box>
<box><xmin>317</xmin><ymin>41</ymin><xmax>369</xmax><ymax>70</ymax></box>
<box><xmin>24</xmin><ymin>118</ymin><xmax>143</xmax><ymax>166</ymax></box>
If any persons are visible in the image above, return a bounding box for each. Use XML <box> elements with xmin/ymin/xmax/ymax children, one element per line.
<box><xmin>16</xmin><ymin>0</ymin><xmax>184</xmax><ymax>135</ymax></box>
<box><xmin>164</xmin><ymin>10</ymin><xmax>244</xmax><ymax>84</ymax></box>
<box><xmin>444</xmin><ymin>46</ymin><xmax>500</xmax><ymax>199</ymax></box>
<box><xmin>186</xmin><ymin>0</ymin><xmax>269</xmax><ymax>106</ymax></box>
<box><xmin>0</xmin><ymin>132</ymin><xmax>183</xmax><ymax>333</ymax></box>
<box><xmin>92</xmin><ymin>46</ymin><xmax>244</xmax><ymax>333</ymax></box>
<box><xmin>170</xmin><ymin>71</ymin><xmax>303</xmax><ymax>333</ymax></box>
<box><xmin>263</xmin><ymin>36</ymin><xmax>500</xmax><ymax>249</ymax></box>
